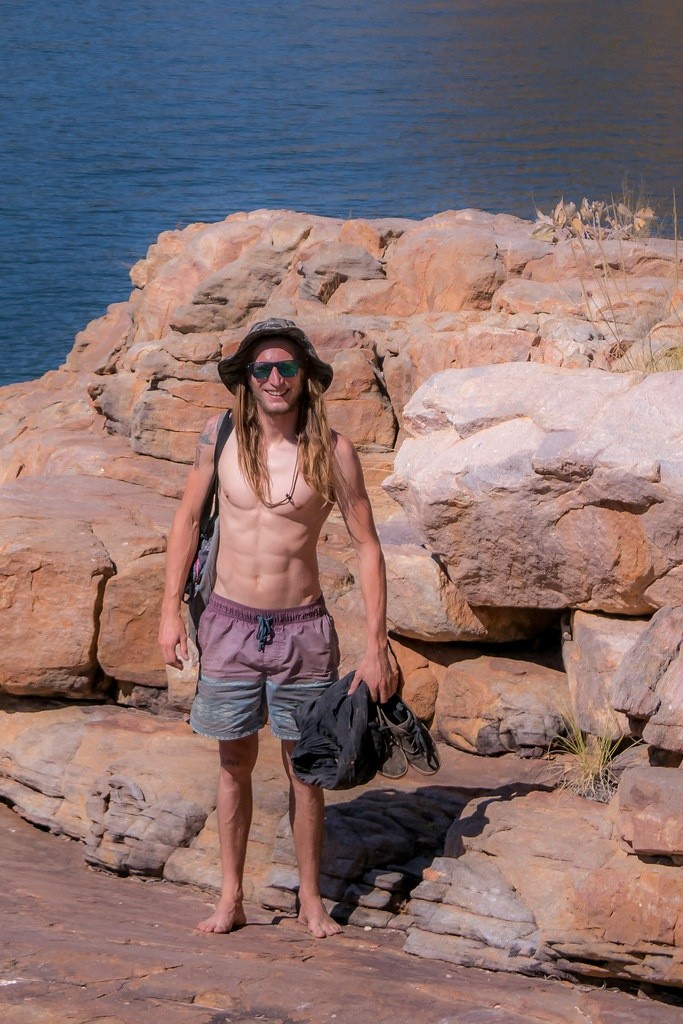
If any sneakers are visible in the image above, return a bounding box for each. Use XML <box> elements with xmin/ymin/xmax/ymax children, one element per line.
<box><xmin>368</xmin><ymin>694</ymin><xmax>442</xmax><ymax>779</ymax></box>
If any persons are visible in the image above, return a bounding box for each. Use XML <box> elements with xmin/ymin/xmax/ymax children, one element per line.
<box><xmin>158</xmin><ymin>317</ymin><xmax>398</xmax><ymax>938</ymax></box>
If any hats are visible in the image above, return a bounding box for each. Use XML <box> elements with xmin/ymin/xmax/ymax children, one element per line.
<box><xmin>218</xmin><ymin>318</ymin><xmax>333</xmax><ymax>398</ymax></box>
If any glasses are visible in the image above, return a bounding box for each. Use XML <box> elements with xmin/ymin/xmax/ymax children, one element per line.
<box><xmin>246</xmin><ymin>359</ymin><xmax>304</xmax><ymax>378</ymax></box>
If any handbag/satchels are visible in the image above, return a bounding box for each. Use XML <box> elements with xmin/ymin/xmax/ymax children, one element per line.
<box><xmin>181</xmin><ymin>409</ymin><xmax>236</xmax><ymax>629</ymax></box>
